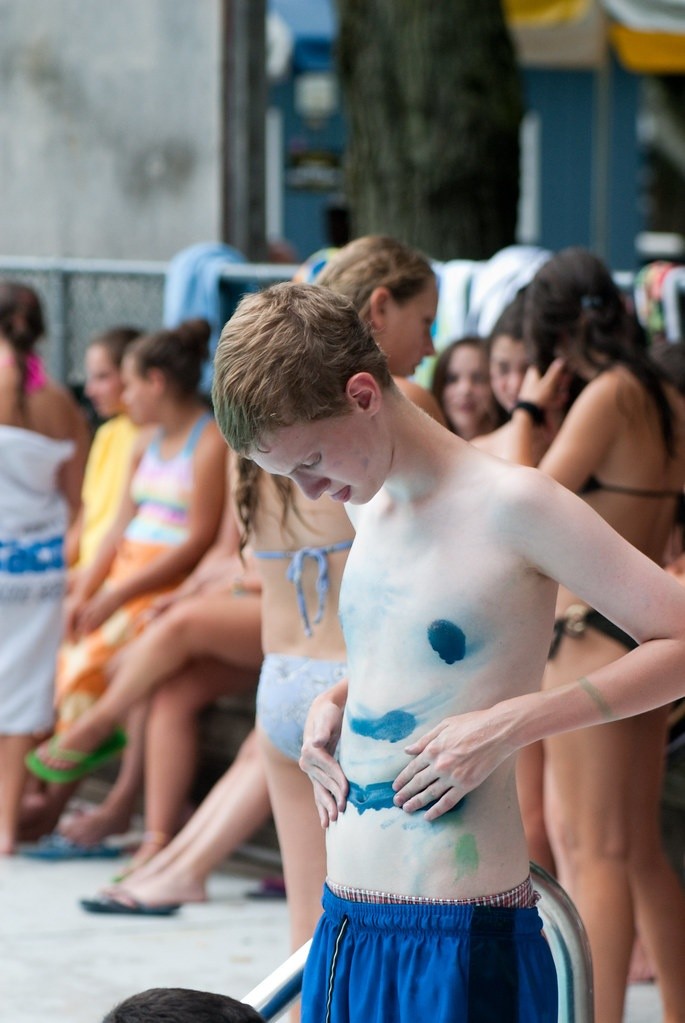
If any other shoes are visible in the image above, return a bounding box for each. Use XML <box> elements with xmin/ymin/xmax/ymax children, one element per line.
<box><xmin>247</xmin><ymin>876</ymin><xmax>287</xmax><ymax>898</ymax></box>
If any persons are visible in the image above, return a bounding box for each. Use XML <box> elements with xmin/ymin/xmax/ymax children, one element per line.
<box><xmin>234</xmin><ymin>234</ymin><xmax>451</xmax><ymax>1023</ymax></box>
<box><xmin>511</xmin><ymin>248</ymin><xmax>685</xmax><ymax>1023</ymax></box>
<box><xmin>0</xmin><ymin>280</ymin><xmax>685</xmax><ymax>987</ymax></box>
<box><xmin>212</xmin><ymin>281</ymin><xmax>685</xmax><ymax>1023</ymax></box>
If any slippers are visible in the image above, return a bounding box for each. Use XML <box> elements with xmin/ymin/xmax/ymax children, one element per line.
<box><xmin>80</xmin><ymin>891</ymin><xmax>182</xmax><ymax>915</ymax></box>
<box><xmin>18</xmin><ymin>830</ymin><xmax>123</xmax><ymax>860</ymax></box>
<box><xmin>23</xmin><ymin>719</ymin><xmax>127</xmax><ymax>784</ymax></box>
